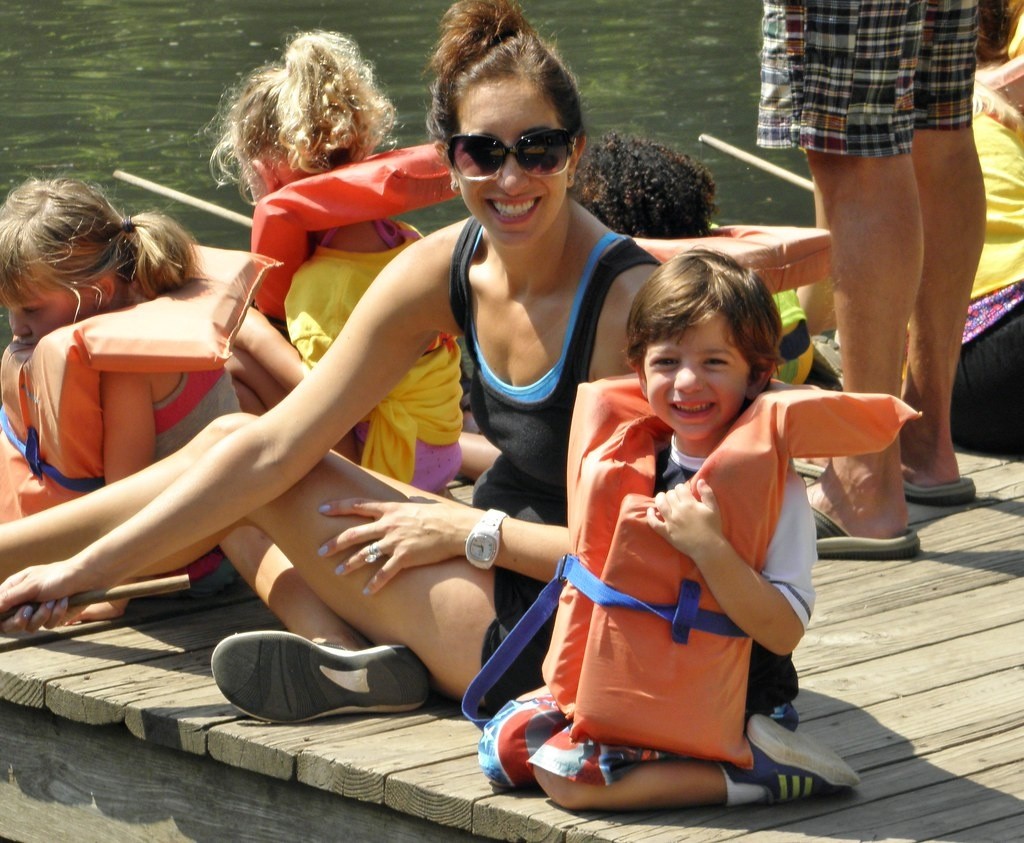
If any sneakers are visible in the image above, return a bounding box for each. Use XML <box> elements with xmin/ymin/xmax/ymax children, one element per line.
<box><xmin>211</xmin><ymin>629</ymin><xmax>432</xmax><ymax>723</ymax></box>
<box><xmin>766</xmin><ymin>701</ymin><xmax>800</xmax><ymax>733</ymax></box>
<box><xmin>720</xmin><ymin>713</ymin><xmax>861</xmax><ymax>806</ymax></box>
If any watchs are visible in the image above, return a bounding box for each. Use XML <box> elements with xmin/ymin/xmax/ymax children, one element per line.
<box><xmin>463</xmin><ymin>507</ymin><xmax>511</xmax><ymax>569</ymax></box>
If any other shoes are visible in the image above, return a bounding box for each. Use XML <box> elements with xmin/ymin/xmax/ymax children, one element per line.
<box><xmin>809</xmin><ymin>335</ymin><xmax>847</xmax><ymax>392</ymax></box>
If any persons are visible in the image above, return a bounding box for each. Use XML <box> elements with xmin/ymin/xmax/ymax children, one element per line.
<box><xmin>755</xmin><ymin>0</ymin><xmax>987</xmax><ymax>559</ymax></box>
<box><xmin>948</xmin><ymin>0</ymin><xmax>1024</xmax><ymax>458</ymax></box>
<box><xmin>457</xmin><ymin>130</ymin><xmax>845</xmax><ymax>485</ymax></box>
<box><xmin>223</xmin><ymin>27</ymin><xmax>467</xmax><ymax>501</ymax></box>
<box><xmin>0</xmin><ymin>176</ymin><xmax>246</xmax><ymax>627</ymax></box>
<box><xmin>481</xmin><ymin>246</ymin><xmax>863</xmax><ymax>815</ymax></box>
<box><xmin>1</xmin><ymin>1</ymin><xmax>668</xmax><ymax>722</ymax></box>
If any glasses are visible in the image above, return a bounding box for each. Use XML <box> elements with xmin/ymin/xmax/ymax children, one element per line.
<box><xmin>444</xmin><ymin>129</ymin><xmax>577</xmax><ymax>178</ymax></box>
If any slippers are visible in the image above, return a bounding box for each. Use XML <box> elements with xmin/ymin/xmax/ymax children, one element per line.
<box><xmin>794</xmin><ymin>457</ymin><xmax>977</xmax><ymax>504</ymax></box>
<box><xmin>810</xmin><ymin>498</ymin><xmax>921</xmax><ymax>563</ymax></box>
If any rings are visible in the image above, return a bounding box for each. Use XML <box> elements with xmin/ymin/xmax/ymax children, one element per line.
<box><xmin>364</xmin><ymin>542</ymin><xmax>382</xmax><ymax>563</ymax></box>
